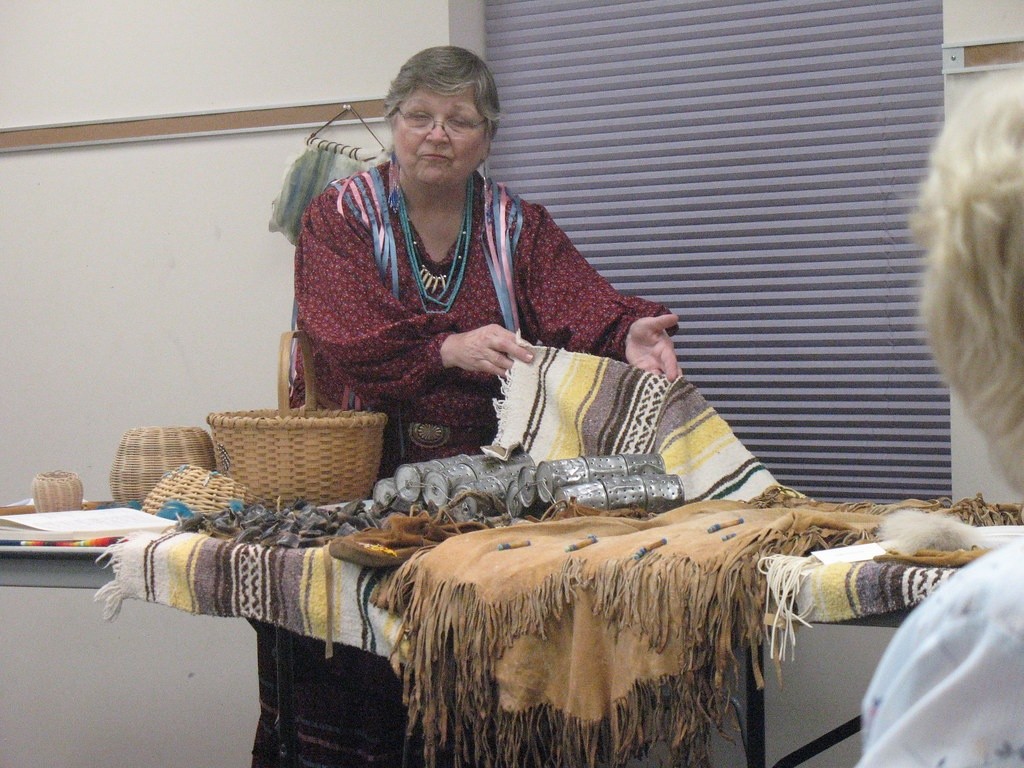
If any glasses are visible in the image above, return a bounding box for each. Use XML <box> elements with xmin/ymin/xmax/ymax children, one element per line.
<box><xmin>397</xmin><ymin>106</ymin><xmax>485</xmax><ymax>134</ymax></box>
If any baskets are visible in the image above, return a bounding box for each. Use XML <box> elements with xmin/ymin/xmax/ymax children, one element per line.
<box><xmin>33</xmin><ymin>470</ymin><xmax>82</xmax><ymax>511</ymax></box>
<box><xmin>208</xmin><ymin>330</ymin><xmax>387</xmax><ymax>512</ymax></box>
<box><xmin>110</xmin><ymin>426</ymin><xmax>215</xmax><ymax>513</ymax></box>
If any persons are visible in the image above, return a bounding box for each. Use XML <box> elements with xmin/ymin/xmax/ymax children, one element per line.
<box><xmin>286</xmin><ymin>45</ymin><xmax>684</xmax><ymax>491</ymax></box>
<box><xmin>854</xmin><ymin>67</ymin><xmax>1024</xmax><ymax>768</ymax></box>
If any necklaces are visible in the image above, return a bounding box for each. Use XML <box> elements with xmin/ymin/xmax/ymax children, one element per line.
<box><xmin>396</xmin><ymin>171</ymin><xmax>473</xmax><ymax>315</ymax></box>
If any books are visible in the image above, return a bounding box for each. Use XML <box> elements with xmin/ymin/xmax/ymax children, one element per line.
<box><xmin>0</xmin><ymin>506</ymin><xmax>179</xmax><ymax>542</ymax></box>
